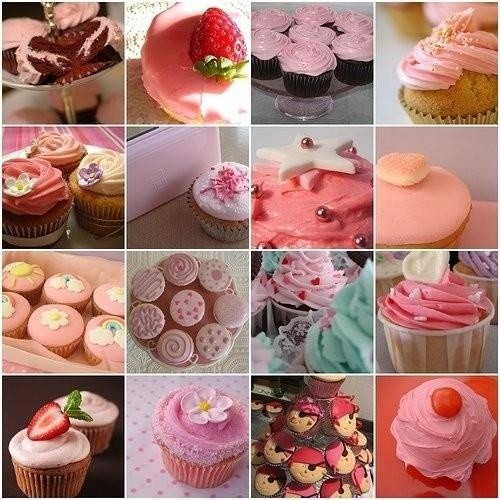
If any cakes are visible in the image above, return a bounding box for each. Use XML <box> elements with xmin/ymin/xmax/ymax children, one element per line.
<box><xmin>320</xmin><ymin>478</ymin><xmax>351</xmax><ymax>498</ymax></box>
<box><xmin>396</xmin><ymin>5</ymin><xmax>498</xmax><ymax>121</ymax></box>
<box><xmin>29</xmin><ymin>304</ymin><xmax>84</xmax><ymax>357</ymax></box>
<box><xmin>272</xmin><ymin>250</ymin><xmax>346</xmax><ymax>333</ymax></box>
<box><xmin>250</xmin><ymin>9</ymin><xmax>291</xmax><ymax>33</ymax></box>
<box><xmin>375</xmin><ymin>254</ymin><xmax>496</xmax><ymax>375</ymax></box>
<box><xmin>335</xmin><ymin>10</ymin><xmax>373</xmax><ymax>35</ymax></box>
<box><xmin>2</xmin><ymin>158</ymin><xmax>74</xmax><ymax>247</ymax></box>
<box><xmin>163</xmin><ymin>252</ymin><xmax>198</xmax><ymax>286</ymax></box>
<box><xmin>280</xmin><ymin>41</ymin><xmax>334</xmax><ymax>98</ymax></box>
<box><xmin>262</xmin><ymin>432</ymin><xmax>295</xmax><ymax>465</ymax></box>
<box><xmin>213</xmin><ymin>294</ymin><xmax>249</xmax><ymax>327</ymax></box>
<box><xmin>251</xmin><ymin>399</ymin><xmax>263</xmax><ymax>414</ymax></box>
<box><xmin>293</xmin><ymin>4</ymin><xmax>335</xmax><ymax>27</ymax></box>
<box><xmin>250</xmin><ymin>29</ymin><xmax>290</xmax><ymax>80</ymax></box>
<box><xmin>288</xmin><ymin>404</ymin><xmax>320</xmax><ymax>437</ymax></box>
<box><xmin>9</xmin><ymin>427</ymin><xmax>92</xmax><ymax>495</ymax></box>
<box><xmin>354</xmin><ymin>448</ymin><xmax>372</xmax><ymax>464</ymax></box>
<box><xmin>184</xmin><ymin>162</ymin><xmax>250</xmax><ymax>242</ymax></box>
<box><xmin>352</xmin><ymin>464</ymin><xmax>371</xmax><ymax>492</ymax></box>
<box><xmin>377</xmin><ymin>255</ymin><xmax>406</xmax><ymax>295</ymax></box>
<box><xmin>2</xmin><ymin>1</ymin><xmax>123</xmax><ymax>85</ymax></box>
<box><xmin>452</xmin><ymin>252</ymin><xmax>496</xmax><ymax>329</ymax></box>
<box><xmin>375</xmin><ymin>153</ymin><xmax>474</xmax><ymax>250</ymax></box>
<box><xmin>85</xmin><ymin>315</ymin><xmax>126</xmax><ymax>365</ymax></box>
<box><xmin>327</xmin><ymin>441</ymin><xmax>355</xmax><ymax>475</ymax></box>
<box><xmin>287</xmin><ymin>23</ymin><xmax>334</xmax><ymax>44</ymax></box>
<box><xmin>286</xmin><ymin>482</ymin><xmax>318</xmax><ymax>497</ymax></box>
<box><xmin>3</xmin><ymin>261</ymin><xmax>43</xmax><ymax>303</ymax></box>
<box><xmin>391</xmin><ymin>379</ymin><xmax>498</xmax><ymax>483</ymax></box>
<box><xmin>2</xmin><ymin>293</ymin><xmax>31</xmax><ymax>337</ymax></box>
<box><xmin>55</xmin><ymin>391</ymin><xmax>117</xmax><ymax>453</ymax></box>
<box><xmin>250</xmin><ymin>134</ymin><xmax>374</xmax><ymax>251</ymax></box>
<box><xmin>290</xmin><ymin>448</ymin><xmax>325</xmax><ymax>483</ymax></box>
<box><xmin>337</xmin><ymin>34</ymin><xmax>373</xmax><ymax>85</ymax></box>
<box><xmin>133</xmin><ymin>269</ymin><xmax>165</xmax><ymax>302</ymax></box>
<box><xmin>129</xmin><ymin>305</ymin><xmax>163</xmax><ymax>340</ymax></box>
<box><xmin>197</xmin><ymin>259</ymin><xmax>233</xmax><ymax>291</ymax></box>
<box><xmin>42</xmin><ymin>272</ymin><xmax>93</xmax><ymax>305</ymax></box>
<box><xmin>320</xmin><ymin>400</ymin><xmax>355</xmax><ymax>440</ymax></box>
<box><xmin>352</xmin><ymin>430</ymin><xmax>367</xmax><ymax>449</ymax></box>
<box><xmin>141</xmin><ymin>2</ymin><xmax>251</xmax><ymax>124</ymax></box>
<box><xmin>170</xmin><ymin>288</ymin><xmax>206</xmax><ymax>326</ymax></box>
<box><xmin>157</xmin><ymin>329</ymin><xmax>194</xmax><ymax>365</ymax></box>
<box><xmin>70</xmin><ymin>150</ymin><xmax>124</xmax><ymax>237</ymax></box>
<box><xmin>305</xmin><ymin>259</ymin><xmax>374</xmax><ymax>373</ymax></box>
<box><xmin>252</xmin><ymin>252</ymin><xmax>268</xmax><ymax>338</ymax></box>
<box><xmin>254</xmin><ymin>464</ymin><xmax>286</xmax><ymax>496</ymax></box>
<box><xmin>306</xmin><ymin>377</ymin><xmax>345</xmax><ymax>397</ymax></box>
<box><xmin>92</xmin><ymin>285</ymin><xmax>124</xmax><ymax>314</ymax></box>
<box><xmin>265</xmin><ymin>402</ymin><xmax>283</xmax><ymax>417</ymax></box>
<box><xmin>152</xmin><ymin>385</ymin><xmax>250</xmax><ymax>488</ymax></box>
<box><xmin>24</xmin><ymin>131</ymin><xmax>86</xmax><ymax>175</ymax></box>
<box><xmin>196</xmin><ymin>324</ymin><xmax>232</xmax><ymax>360</ymax></box>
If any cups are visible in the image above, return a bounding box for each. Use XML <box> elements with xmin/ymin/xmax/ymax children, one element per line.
<box><xmin>253</xmin><ymin>376</ymin><xmax>373</xmax><ymax>498</ymax></box>
<box><xmin>2</xmin><ymin>125</ymin><xmax>124</xmax><ymax>498</ymax></box>
<box><xmin>251</xmin><ymin>2</ymin><xmax>498</xmax><ymax>374</ymax></box>
<box><xmin>187</xmin><ymin>173</ymin><xmax>250</xmax><ymax>243</ymax></box>
<box><xmin>50</xmin><ymin>104</ymin><xmax>98</xmax><ymax>125</ymax></box>
<box><xmin>153</xmin><ymin>430</ymin><xmax>245</xmax><ymax>488</ymax></box>
